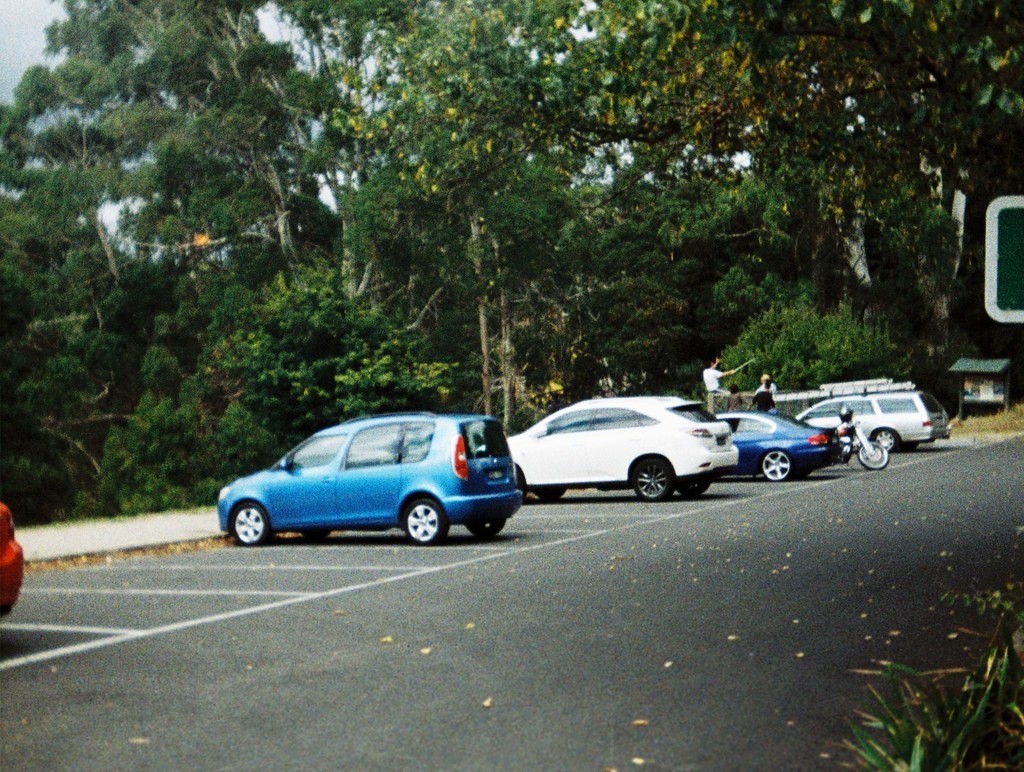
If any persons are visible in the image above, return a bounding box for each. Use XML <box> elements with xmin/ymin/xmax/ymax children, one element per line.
<box><xmin>754</xmin><ymin>374</ymin><xmax>776</xmax><ymax>396</ymax></box>
<box><xmin>702</xmin><ymin>357</ymin><xmax>734</xmax><ymax>408</ymax></box>
<box><xmin>726</xmin><ymin>384</ymin><xmax>743</xmax><ymax>411</ymax></box>
<box><xmin>746</xmin><ymin>382</ymin><xmax>775</xmax><ymax>412</ymax></box>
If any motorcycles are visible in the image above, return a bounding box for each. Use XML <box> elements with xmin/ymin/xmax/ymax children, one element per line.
<box><xmin>828</xmin><ymin>404</ymin><xmax>890</xmax><ymax>470</ymax></box>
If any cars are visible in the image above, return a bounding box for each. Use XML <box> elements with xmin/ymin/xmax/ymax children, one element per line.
<box><xmin>716</xmin><ymin>412</ymin><xmax>839</xmax><ymax>482</ymax></box>
<box><xmin>796</xmin><ymin>390</ymin><xmax>951</xmax><ymax>452</ymax></box>
<box><xmin>477</xmin><ymin>396</ymin><xmax>740</xmax><ymax>502</ymax></box>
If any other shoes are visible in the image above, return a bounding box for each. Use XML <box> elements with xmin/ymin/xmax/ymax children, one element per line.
<box><xmin>716</xmin><ymin>402</ymin><xmax>721</xmax><ymax>409</ymax></box>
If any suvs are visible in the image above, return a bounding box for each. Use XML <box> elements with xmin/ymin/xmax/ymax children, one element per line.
<box><xmin>217</xmin><ymin>409</ymin><xmax>523</xmax><ymax>544</ymax></box>
